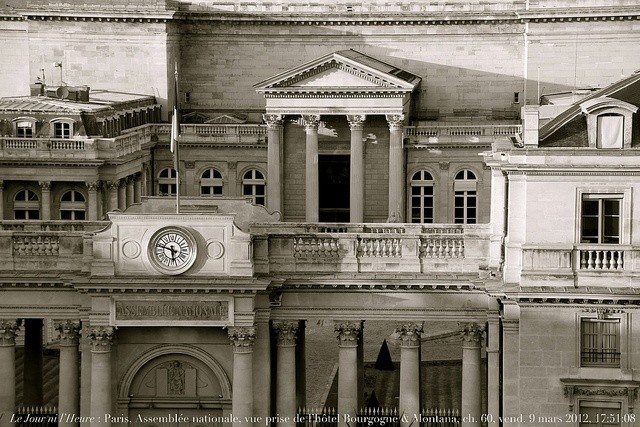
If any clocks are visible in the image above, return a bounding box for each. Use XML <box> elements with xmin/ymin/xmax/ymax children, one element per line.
<box><xmin>147</xmin><ymin>225</ymin><xmax>198</xmax><ymax>275</ymax></box>
